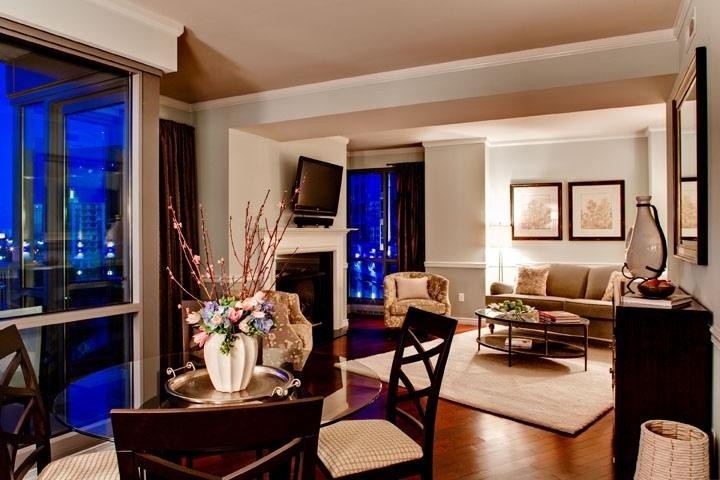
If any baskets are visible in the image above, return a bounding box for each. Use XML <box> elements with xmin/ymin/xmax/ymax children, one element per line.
<box><xmin>633</xmin><ymin>420</ymin><xmax>710</xmax><ymax>480</ymax></box>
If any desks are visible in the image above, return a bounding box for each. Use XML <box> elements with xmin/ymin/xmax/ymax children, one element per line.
<box><xmin>50</xmin><ymin>352</ymin><xmax>381</xmax><ymax>440</ymax></box>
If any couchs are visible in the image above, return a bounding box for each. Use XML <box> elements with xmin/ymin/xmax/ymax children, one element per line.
<box><xmin>484</xmin><ymin>264</ymin><xmax>632</xmax><ymax>348</ymax></box>
<box><xmin>257</xmin><ymin>290</ymin><xmax>313</xmax><ymax>372</ymax></box>
<box><xmin>383</xmin><ymin>271</ymin><xmax>452</xmax><ymax>328</ymax></box>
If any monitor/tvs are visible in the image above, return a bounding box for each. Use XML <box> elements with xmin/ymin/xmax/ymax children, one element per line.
<box><xmin>293</xmin><ymin>156</ymin><xmax>344</xmax><ymax>216</ymax></box>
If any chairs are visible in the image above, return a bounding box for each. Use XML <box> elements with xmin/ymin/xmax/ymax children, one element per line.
<box><xmin>181</xmin><ymin>301</ymin><xmax>217</xmax><ymax>371</ymax></box>
<box><xmin>294</xmin><ymin>306</ymin><xmax>457</xmax><ymax>480</ymax></box>
<box><xmin>0</xmin><ymin>324</ymin><xmax>120</xmax><ymax>480</ymax></box>
<box><xmin>110</xmin><ymin>397</ymin><xmax>325</xmax><ymax>480</ymax></box>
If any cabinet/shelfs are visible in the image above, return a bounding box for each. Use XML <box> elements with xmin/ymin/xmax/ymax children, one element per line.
<box><xmin>614</xmin><ymin>288</ymin><xmax>714</xmax><ymax>480</ymax></box>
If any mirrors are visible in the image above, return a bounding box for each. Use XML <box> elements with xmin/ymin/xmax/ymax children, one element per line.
<box><xmin>671</xmin><ymin>47</ymin><xmax>707</xmax><ymax>267</ymax></box>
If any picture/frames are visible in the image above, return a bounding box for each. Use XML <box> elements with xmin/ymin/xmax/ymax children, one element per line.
<box><xmin>568</xmin><ymin>180</ymin><xmax>626</xmax><ymax>241</ymax></box>
<box><xmin>510</xmin><ymin>182</ymin><xmax>562</xmax><ymax>240</ymax></box>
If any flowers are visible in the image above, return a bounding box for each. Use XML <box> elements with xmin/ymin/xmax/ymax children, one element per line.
<box><xmin>165</xmin><ymin>188</ymin><xmax>299</xmax><ymax>355</ymax></box>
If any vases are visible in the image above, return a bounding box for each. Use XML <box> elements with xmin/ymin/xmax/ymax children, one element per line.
<box><xmin>204</xmin><ymin>333</ymin><xmax>255</xmax><ymax>394</ymax></box>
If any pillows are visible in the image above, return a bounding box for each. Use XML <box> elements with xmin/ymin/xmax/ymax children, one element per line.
<box><xmin>395</xmin><ymin>277</ymin><xmax>429</xmax><ymax>300</ymax></box>
<box><xmin>602</xmin><ymin>271</ymin><xmax>630</xmax><ymax>303</ymax></box>
<box><xmin>515</xmin><ymin>265</ymin><xmax>550</xmax><ymax>296</ymax></box>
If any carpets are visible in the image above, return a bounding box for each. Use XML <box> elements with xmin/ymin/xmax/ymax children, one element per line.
<box><xmin>334</xmin><ymin>324</ymin><xmax>613</xmax><ymax>437</ymax></box>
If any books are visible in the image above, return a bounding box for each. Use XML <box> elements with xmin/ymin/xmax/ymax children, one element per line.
<box><xmin>505</xmin><ymin>337</ymin><xmax>532</xmax><ymax>349</ymax></box>
<box><xmin>539</xmin><ymin>310</ymin><xmax>581</xmax><ymax>325</ymax></box>
<box><xmin>621</xmin><ymin>295</ymin><xmax>693</xmax><ymax>309</ymax></box>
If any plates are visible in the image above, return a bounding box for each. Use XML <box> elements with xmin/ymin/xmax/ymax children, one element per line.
<box><xmin>637</xmin><ymin>283</ymin><xmax>676</xmax><ymax>299</ymax></box>
<box><xmin>163</xmin><ymin>361</ymin><xmax>301</xmax><ymax>405</ymax></box>
<box><xmin>487</xmin><ymin>302</ymin><xmax>535</xmax><ymax>314</ymax></box>
